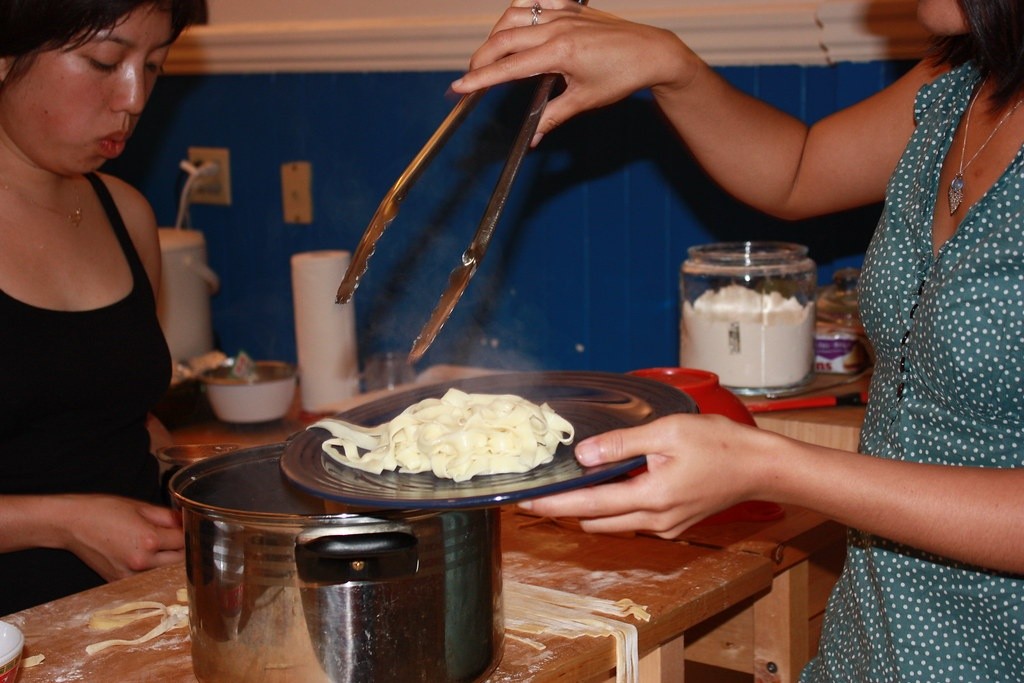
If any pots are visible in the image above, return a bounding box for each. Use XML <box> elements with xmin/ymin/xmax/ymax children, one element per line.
<box><xmin>167</xmin><ymin>440</ymin><xmax>506</xmax><ymax>682</ymax></box>
<box><xmin>175</xmin><ymin>356</ymin><xmax>300</xmax><ymax>425</ymax></box>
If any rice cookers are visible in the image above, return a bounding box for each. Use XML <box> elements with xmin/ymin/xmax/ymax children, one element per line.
<box><xmin>154</xmin><ymin>227</ymin><xmax>220</xmax><ymax>362</ymax></box>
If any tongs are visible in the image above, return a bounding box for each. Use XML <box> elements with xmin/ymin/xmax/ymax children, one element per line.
<box><xmin>337</xmin><ymin>0</ymin><xmax>593</xmax><ymax>369</ymax></box>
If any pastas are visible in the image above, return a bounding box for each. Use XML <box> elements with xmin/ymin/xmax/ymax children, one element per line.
<box><xmin>306</xmin><ymin>388</ymin><xmax>574</xmax><ymax>482</ymax></box>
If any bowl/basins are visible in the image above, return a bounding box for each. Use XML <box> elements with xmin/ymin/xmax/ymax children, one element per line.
<box><xmin>0</xmin><ymin>620</ymin><xmax>27</xmax><ymax>683</ymax></box>
<box><xmin>625</xmin><ymin>367</ymin><xmax>758</xmax><ymax>428</ymax></box>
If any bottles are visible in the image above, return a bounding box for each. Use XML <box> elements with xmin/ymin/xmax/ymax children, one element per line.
<box><xmin>678</xmin><ymin>239</ymin><xmax>819</xmax><ymax>398</ymax></box>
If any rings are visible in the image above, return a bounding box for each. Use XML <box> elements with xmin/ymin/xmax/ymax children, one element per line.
<box><xmin>531</xmin><ymin>2</ymin><xmax>543</xmax><ymax>26</ymax></box>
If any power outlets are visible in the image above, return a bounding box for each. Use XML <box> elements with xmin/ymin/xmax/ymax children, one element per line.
<box><xmin>188</xmin><ymin>146</ymin><xmax>231</xmax><ymax>205</ymax></box>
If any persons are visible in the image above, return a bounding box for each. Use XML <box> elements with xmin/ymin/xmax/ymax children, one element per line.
<box><xmin>452</xmin><ymin>0</ymin><xmax>1024</xmax><ymax>683</ymax></box>
<box><xmin>0</xmin><ymin>0</ymin><xmax>185</xmax><ymax>617</ymax></box>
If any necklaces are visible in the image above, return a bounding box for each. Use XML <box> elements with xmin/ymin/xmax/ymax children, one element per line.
<box><xmin>0</xmin><ymin>179</ymin><xmax>82</xmax><ymax>228</ymax></box>
<box><xmin>947</xmin><ymin>71</ymin><xmax>1024</xmax><ymax>214</ymax></box>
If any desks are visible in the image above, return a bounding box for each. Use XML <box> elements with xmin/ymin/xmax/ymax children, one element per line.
<box><xmin>0</xmin><ymin>509</ymin><xmax>774</xmax><ymax>683</ymax></box>
<box><xmin>147</xmin><ymin>376</ymin><xmax>873</xmax><ymax>683</ymax></box>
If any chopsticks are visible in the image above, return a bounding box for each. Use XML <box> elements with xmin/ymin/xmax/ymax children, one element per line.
<box><xmin>736</xmin><ymin>385</ymin><xmax>873</xmax><ymax>415</ymax></box>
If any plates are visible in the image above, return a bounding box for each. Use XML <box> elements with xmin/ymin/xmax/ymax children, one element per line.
<box><xmin>279</xmin><ymin>369</ymin><xmax>700</xmax><ymax>509</ymax></box>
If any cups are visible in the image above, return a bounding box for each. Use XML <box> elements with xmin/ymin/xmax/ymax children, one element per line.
<box><xmin>363</xmin><ymin>354</ymin><xmax>416</xmax><ymax>392</ymax></box>
